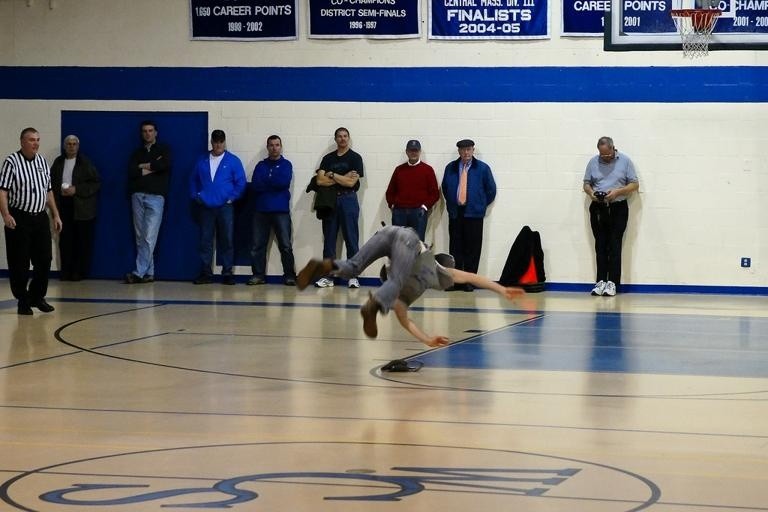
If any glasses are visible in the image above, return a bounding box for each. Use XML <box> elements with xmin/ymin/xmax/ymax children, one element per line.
<box><xmin>601</xmin><ymin>151</ymin><xmax>614</xmax><ymax>158</ymax></box>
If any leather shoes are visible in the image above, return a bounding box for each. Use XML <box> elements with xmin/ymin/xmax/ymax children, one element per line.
<box><xmin>222</xmin><ymin>276</ymin><xmax>236</xmax><ymax>284</ymax></box>
<box><xmin>31</xmin><ymin>298</ymin><xmax>54</xmax><ymax>312</ymax></box>
<box><xmin>193</xmin><ymin>275</ymin><xmax>213</xmax><ymax>284</ymax></box>
<box><xmin>18</xmin><ymin>306</ymin><xmax>33</xmax><ymax>315</ymax></box>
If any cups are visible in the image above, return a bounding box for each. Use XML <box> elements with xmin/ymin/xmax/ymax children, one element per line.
<box><xmin>61</xmin><ymin>182</ymin><xmax>70</xmax><ymax>196</ymax></box>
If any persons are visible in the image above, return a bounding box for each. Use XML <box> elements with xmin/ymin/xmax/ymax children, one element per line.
<box><xmin>442</xmin><ymin>140</ymin><xmax>496</xmax><ymax>292</ymax></box>
<box><xmin>295</xmin><ymin>221</ymin><xmax>526</xmax><ymax>348</ymax></box>
<box><xmin>50</xmin><ymin>135</ymin><xmax>102</xmax><ymax>281</ymax></box>
<box><xmin>122</xmin><ymin>121</ymin><xmax>172</xmax><ymax>284</ymax></box>
<box><xmin>2</xmin><ymin>128</ymin><xmax>62</xmax><ymax>316</ymax></box>
<box><xmin>583</xmin><ymin>136</ymin><xmax>639</xmax><ymax>296</ymax></box>
<box><xmin>306</xmin><ymin>128</ymin><xmax>363</xmax><ymax>288</ymax></box>
<box><xmin>185</xmin><ymin>130</ymin><xmax>247</xmax><ymax>284</ymax></box>
<box><xmin>246</xmin><ymin>136</ymin><xmax>296</xmax><ymax>286</ymax></box>
<box><xmin>386</xmin><ymin>141</ymin><xmax>440</xmax><ymax>242</ymax></box>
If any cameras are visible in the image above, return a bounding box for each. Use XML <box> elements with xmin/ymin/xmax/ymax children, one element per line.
<box><xmin>594</xmin><ymin>192</ymin><xmax>607</xmax><ymax>201</ymax></box>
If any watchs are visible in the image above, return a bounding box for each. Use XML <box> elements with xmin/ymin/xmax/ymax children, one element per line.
<box><xmin>329</xmin><ymin>172</ymin><xmax>335</xmax><ymax>179</ymax></box>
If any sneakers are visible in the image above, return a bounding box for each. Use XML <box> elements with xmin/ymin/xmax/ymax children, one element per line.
<box><xmin>71</xmin><ymin>275</ymin><xmax>81</xmax><ymax>281</ymax></box>
<box><xmin>140</xmin><ymin>276</ymin><xmax>153</xmax><ymax>283</ymax></box>
<box><xmin>602</xmin><ymin>281</ymin><xmax>617</xmax><ymax>297</ymax></box>
<box><xmin>314</xmin><ymin>278</ymin><xmax>334</xmax><ymax>288</ymax></box>
<box><xmin>444</xmin><ymin>286</ymin><xmax>462</xmax><ymax>291</ymax></box>
<box><xmin>126</xmin><ymin>273</ymin><xmax>139</xmax><ymax>283</ymax></box>
<box><xmin>360</xmin><ymin>293</ymin><xmax>381</xmax><ymax>339</ymax></box>
<box><xmin>247</xmin><ymin>276</ymin><xmax>265</xmax><ymax>285</ymax></box>
<box><xmin>60</xmin><ymin>275</ymin><xmax>69</xmax><ymax>281</ymax></box>
<box><xmin>463</xmin><ymin>282</ymin><xmax>474</xmax><ymax>292</ymax></box>
<box><xmin>285</xmin><ymin>275</ymin><xmax>296</xmax><ymax>285</ymax></box>
<box><xmin>348</xmin><ymin>278</ymin><xmax>360</xmax><ymax>288</ymax></box>
<box><xmin>591</xmin><ymin>280</ymin><xmax>606</xmax><ymax>295</ymax></box>
<box><xmin>296</xmin><ymin>258</ymin><xmax>332</xmax><ymax>291</ymax></box>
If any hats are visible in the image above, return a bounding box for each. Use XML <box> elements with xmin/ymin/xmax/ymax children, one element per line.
<box><xmin>407</xmin><ymin>140</ymin><xmax>421</xmax><ymax>151</ymax></box>
<box><xmin>212</xmin><ymin>130</ymin><xmax>225</xmax><ymax>141</ymax></box>
<box><xmin>457</xmin><ymin>140</ymin><xmax>475</xmax><ymax>147</ymax></box>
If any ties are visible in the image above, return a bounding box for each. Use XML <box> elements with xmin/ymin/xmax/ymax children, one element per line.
<box><xmin>458</xmin><ymin>164</ymin><xmax>467</xmax><ymax>205</ymax></box>
